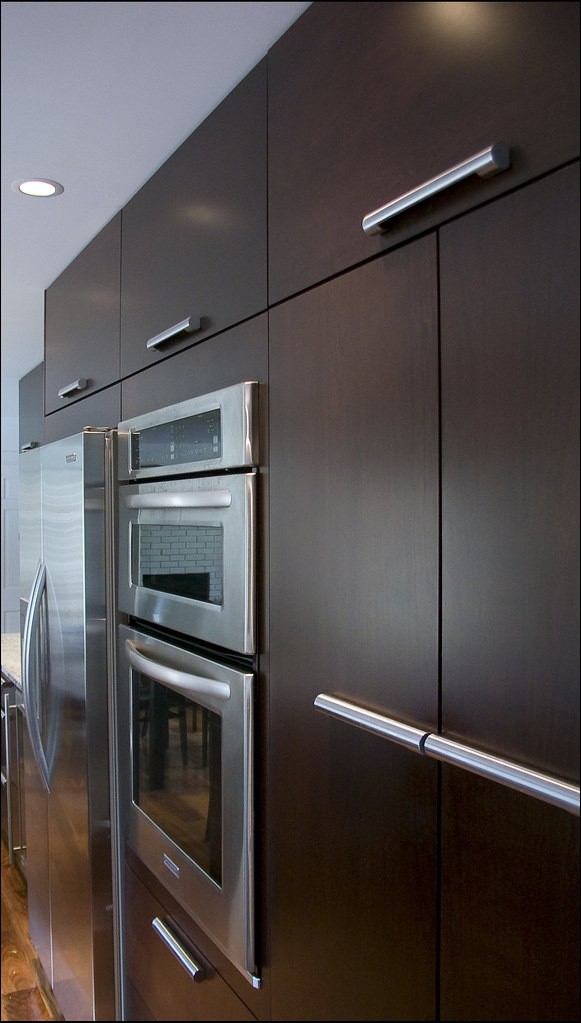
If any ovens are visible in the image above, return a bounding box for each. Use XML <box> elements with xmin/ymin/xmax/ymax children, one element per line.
<box><xmin>113</xmin><ymin>382</ymin><xmax>262</xmax><ymax>971</ymax></box>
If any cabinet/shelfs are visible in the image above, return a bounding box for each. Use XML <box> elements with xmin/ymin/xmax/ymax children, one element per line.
<box><xmin>19</xmin><ymin>55</ymin><xmax>268</xmax><ymax>454</ymax></box>
<box><xmin>259</xmin><ymin>0</ymin><xmax>581</xmax><ymax>1023</ymax></box>
<box><xmin>118</xmin><ymin>847</ymin><xmax>270</xmax><ymax>1023</ymax></box>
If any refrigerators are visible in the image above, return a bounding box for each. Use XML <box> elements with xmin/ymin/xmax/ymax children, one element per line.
<box><xmin>17</xmin><ymin>425</ymin><xmax>115</xmax><ymax>1021</ymax></box>
